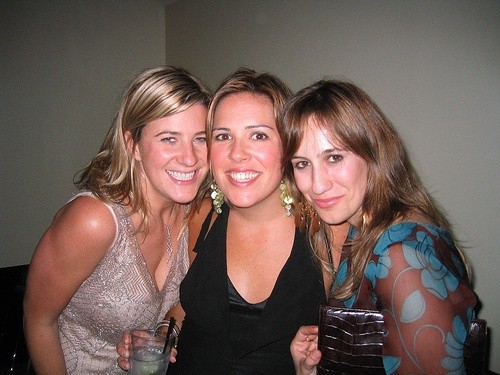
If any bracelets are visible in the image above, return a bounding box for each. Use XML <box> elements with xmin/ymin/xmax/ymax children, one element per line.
<box><xmin>153</xmin><ymin>319</ymin><xmax>182</xmax><ymax>348</ymax></box>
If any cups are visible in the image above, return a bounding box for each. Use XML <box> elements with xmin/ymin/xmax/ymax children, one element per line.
<box><xmin>128</xmin><ymin>327</ymin><xmax>174</xmax><ymax>375</ymax></box>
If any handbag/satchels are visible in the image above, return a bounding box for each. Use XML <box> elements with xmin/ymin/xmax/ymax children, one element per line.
<box><xmin>317</xmin><ymin>306</ymin><xmax>487</xmax><ymax>375</ymax></box>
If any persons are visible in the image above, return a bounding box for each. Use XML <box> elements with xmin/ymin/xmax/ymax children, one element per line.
<box><xmin>116</xmin><ymin>67</ymin><xmax>350</xmax><ymax>375</ymax></box>
<box><xmin>274</xmin><ymin>79</ymin><xmax>478</xmax><ymax>375</ymax></box>
<box><xmin>22</xmin><ymin>65</ymin><xmax>215</xmax><ymax>375</ymax></box>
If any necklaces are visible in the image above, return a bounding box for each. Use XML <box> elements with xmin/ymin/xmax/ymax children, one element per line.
<box><xmin>322</xmin><ymin>227</ymin><xmax>335</xmax><ymax>271</ymax></box>
<box><xmin>149</xmin><ymin>202</ymin><xmax>176</xmax><ymax>267</ymax></box>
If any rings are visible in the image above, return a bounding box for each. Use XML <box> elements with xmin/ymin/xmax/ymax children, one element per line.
<box><xmin>307</xmin><ymin>336</ymin><xmax>309</xmax><ymax>342</ymax></box>
